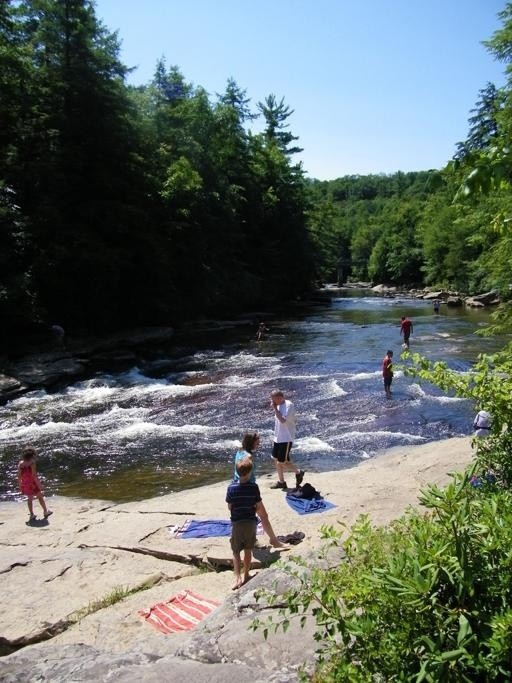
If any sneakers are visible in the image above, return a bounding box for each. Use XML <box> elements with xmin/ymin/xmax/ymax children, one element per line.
<box><xmin>269</xmin><ymin>480</ymin><xmax>287</xmax><ymax>489</ymax></box>
<box><xmin>295</xmin><ymin>469</ymin><xmax>305</xmax><ymax>488</ymax></box>
<box><xmin>44</xmin><ymin>510</ymin><xmax>53</xmax><ymax>518</ymax></box>
<box><xmin>30</xmin><ymin>513</ymin><xmax>37</xmax><ymax>519</ymax></box>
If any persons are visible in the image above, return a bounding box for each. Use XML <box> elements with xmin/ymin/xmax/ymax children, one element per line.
<box><xmin>223</xmin><ymin>460</ymin><xmax>263</xmax><ymax>591</ymax></box>
<box><xmin>381</xmin><ymin>349</ymin><xmax>394</xmax><ymax>395</ymax></box>
<box><xmin>232</xmin><ymin>431</ymin><xmax>293</xmax><ymax>549</ymax></box>
<box><xmin>16</xmin><ymin>446</ymin><xmax>53</xmax><ymax>520</ymax></box>
<box><xmin>434</xmin><ymin>300</ymin><xmax>440</xmax><ymax>314</ymax></box>
<box><xmin>269</xmin><ymin>389</ymin><xmax>304</xmax><ymax>488</ymax></box>
<box><xmin>255</xmin><ymin>322</ymin><xmax>270</xmax><ymax>342</ymax></box>
<box><xmin>399</xmin><ymin>317</ymin><xmax>414</xmax><ymax>348</ymax></box>
